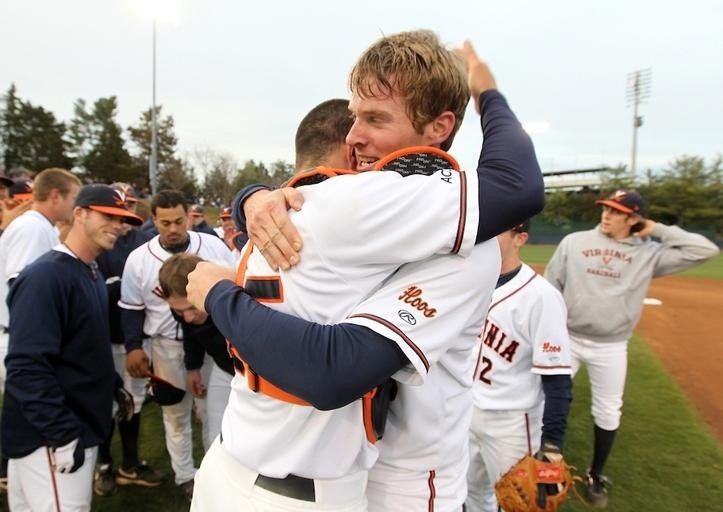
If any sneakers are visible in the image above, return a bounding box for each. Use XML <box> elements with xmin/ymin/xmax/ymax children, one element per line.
<box><xmin>94</xmin><ymin>458</ymin><xmax>118</xmax><ymax>497</ymax></box>
<box><xmin>0</xmin><ymin>477</ymin><xmax>8</xmax><ymax>495</ymax></box>
<box><xmin>179</xmin><ymin>478</ymin><xmax>194</xmax><ymax>502</ymax></box>
<box><xmin>584</xmin><ymin>469</ymin><xmax>614</xmax><ymax>508</ymax></box>
<box><xmin>116</xmin><ymin>461</ymin><xmax>168</xmax><ymax>487</ymax></box>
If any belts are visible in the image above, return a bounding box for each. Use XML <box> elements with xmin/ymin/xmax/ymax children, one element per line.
<box><xmin>220</xmin><ymin>433</ymin><xmax>317</xmax><ymax>502</ymax></box>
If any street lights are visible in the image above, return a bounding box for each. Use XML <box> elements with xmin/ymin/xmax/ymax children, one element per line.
<box><xmin>143</xmin><ymin>8</ymin><xmax>165</xmax><ymax>194</ymax></box>
<box><xmin>624</xmin><ymin>67</ymin><xmax>651</xmax><ymax>180</ymax></box>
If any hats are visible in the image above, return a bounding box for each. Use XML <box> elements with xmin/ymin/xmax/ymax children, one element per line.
<box><xmin>7</xmin><ymin>181</ymin><xmax>34</xmax><ymax>202</ymax></box>
<box><xmin>74</xmin><ymin>184</ymin><xmax>144</xmax><ymax>226</ymax></box>
<box><xmin>110</xmin><ymin>181</ymin><xmax>144</xmax><ymax>205</ymax></box>
<box><xmin>218</xmin><ymin>207</ymin><xmax>231</xmax><ymax>218</ymax></box>
<box><xmin>594</xmin><ymin>188</ymin><xmax>644</xmax><ymax>215</ymax></box>
<box><xmin>188</xmin><ymin>205</ymin><xmax>205</xmax><ymax>218</ymax></box>
<box><xmin>510</xmin><ymin>218</ymin><xmax>531</xmax><ymax>233</ymax></box>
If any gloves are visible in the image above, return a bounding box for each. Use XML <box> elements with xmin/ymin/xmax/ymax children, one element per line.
<box><xmin>532</xmin><ymin>444</ymin><xmax>566</xmax><ymax>509</ymax></box>
<box><xmin>52</xmin><ymin>435</ymin><xmax>85</xmax><ymax>474</ymax></box>
<box><xmin>111</xmin><ymin>387</ymin><xmax>135</xmax><ymax>422</ymax></box>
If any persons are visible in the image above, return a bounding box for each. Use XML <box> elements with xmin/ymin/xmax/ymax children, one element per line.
<box><xmin>461</xmin><ymin>217</ymin><xmax>575</xmax><ymax>512</ymax></box>
<box><xmin>1</xmin><ymin>166</ymin><xmax>243</xmax><ymax>512</ymax></box>
<box><xmin>542</xmin><ymin>190</ymin><xmax>722</xmax><ymax>512</ymax></box>
